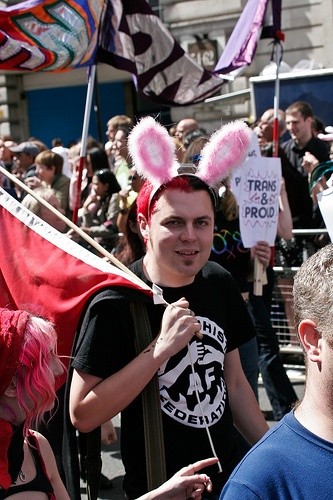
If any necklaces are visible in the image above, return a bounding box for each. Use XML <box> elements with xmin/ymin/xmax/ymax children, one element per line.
<box><xmin>19</xmin><ymin>469</ymin><xmax>27</xmax><ymax>482</ymax></box>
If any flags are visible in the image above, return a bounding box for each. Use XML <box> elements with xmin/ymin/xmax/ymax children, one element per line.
<box><xmin>0</xmin><ymin>0</ymin><xmax>286</xmax><ymax>109</ymax></box>
<box><xmin>0</xmin><ymin>186</ymin><xmax>167</xmax><ymax>391</ymax></box>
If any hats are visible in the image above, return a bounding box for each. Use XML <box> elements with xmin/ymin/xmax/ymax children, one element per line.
<box><xmin>0</xmin><ymin>307</ymin><xmax>31</xmax><ymax>397</ymax></box>
<box><xmin>9</xmin><ymin>142</ymin><xmax>41</xmax><ymax>154</ymax></box>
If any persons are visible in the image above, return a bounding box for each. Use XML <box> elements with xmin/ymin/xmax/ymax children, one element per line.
<box><xmin>0</xmin><ymin>309</ymin><xmax>217</xmax><ymax>500</ymax></box>
<box><xmin>69</xmin><ymin>171</ymin><xmax>270</xmax><ymax>500</ymax></box>
<box><xmin>218</xmin><ymin>243</ymin><xmax>333</xmax><ymax>500</ymax></box>
<box><xmin>1</xmin><ymin>100</ymin><xmax>333</xmax><ymax>489</ymax></box>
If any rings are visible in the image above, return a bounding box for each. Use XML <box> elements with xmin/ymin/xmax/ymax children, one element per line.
<box><xmin>185</xmin><ymin>308</ymin><xmax>193</xmax><ymax>315</ymax></box>
